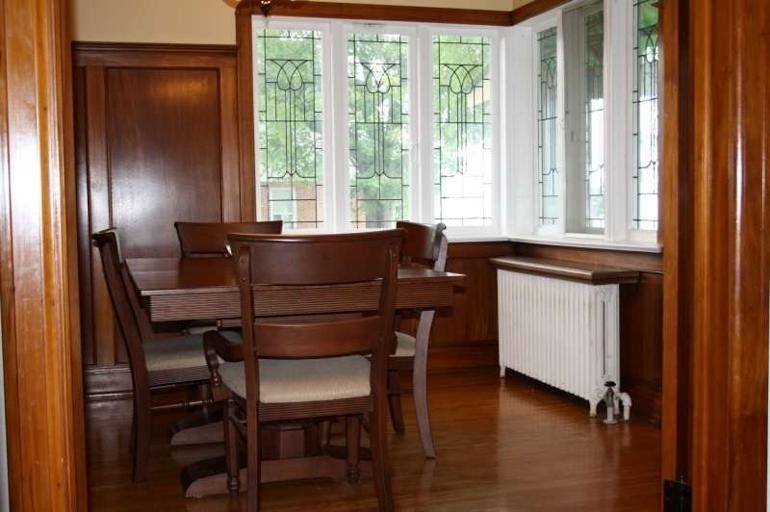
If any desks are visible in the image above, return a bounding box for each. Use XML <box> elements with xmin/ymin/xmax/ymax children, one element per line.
<box><xmin>122</xmin><ymin>255</ymin><xmax>466</xmax><ymax>500</ymax></box>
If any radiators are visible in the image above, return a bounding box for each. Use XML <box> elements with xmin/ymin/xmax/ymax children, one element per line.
<box><xmin>497</xmin><ymin>268</ymin><xmax>622</xmax><ymax>417</ymax></box>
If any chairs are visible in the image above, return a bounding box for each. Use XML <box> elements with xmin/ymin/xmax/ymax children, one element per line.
<box><xmin>201</xmin><ymin>230</ymin><xmax>408</xmax><ymax>512</ymax></box>
<box><xmin>319</xmin><ymin>218</ymin><xmax>452</xmax><ymax>486</ymax></box>
<box><xmin>172</xmin><ymin>219</ymin><xmax>289</xmax><ymax>407</ymax></box>
<box><xmin>91</xmin><ymin>224</ymin><xmax>247</xmax><ymax>485</ymax></box>
